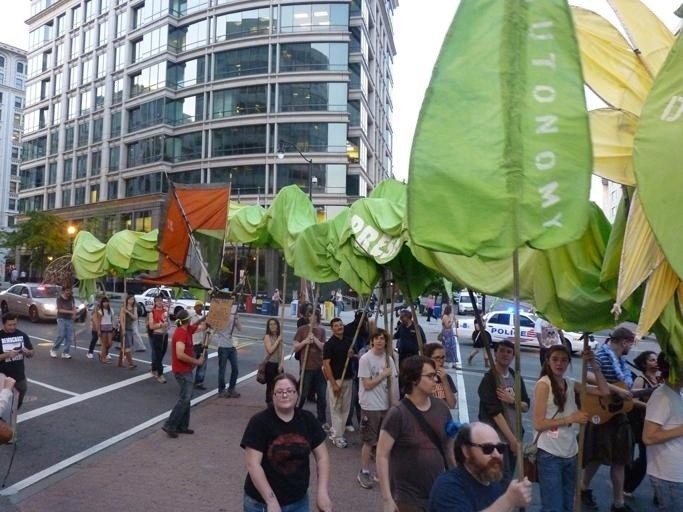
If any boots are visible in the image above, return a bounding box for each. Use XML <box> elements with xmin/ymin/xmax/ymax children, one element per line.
<box><xmin>125</xmin><ymin>350</ymin><xmax>138</xmax><ymax>368</ymax></box>
<box><xmin>117</xmin><ymin>350</ymin><xmax>125</xmax><ymax>369</ymax></box>
<box><xmin>466</xmin><ymin>355</ymin><xmax>474</xmax><ymax>366</ymax></box>
<box><xmin>482</xmin><ymin>358</ymin><xmax>490</xmax><ymax>368</ymax></box>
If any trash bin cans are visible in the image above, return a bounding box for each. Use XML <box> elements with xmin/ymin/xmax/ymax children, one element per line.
<box><xmin>261</xmin><ymin>299</ymin><xmax>272</xmax><ymax>314</ymax></box>
<box><xmin>433</xmin><ymin>306</ymin><xmax>441</xmax><ymax>318</ymax></box>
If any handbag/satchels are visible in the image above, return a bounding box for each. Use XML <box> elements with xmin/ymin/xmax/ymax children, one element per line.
<box><xmin>255</xmin><ymin>360</ymin><xmax>268</xmax><ymax>385</ymax></box>
<box><xmin>110</xmin><ymin>325</ymin><xmax>121</xmax><ymax>343</ymax></box>
<box><xmin>521</xmin><ymin>444</ymin><xmax>538</xmax><ymax>484</ymax></box>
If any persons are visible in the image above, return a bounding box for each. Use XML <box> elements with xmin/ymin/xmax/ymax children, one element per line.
<box><xmin>8</xmin><ymin>266</ymin><xmax>28</xmax><ymax>286</ymax></box>
<box><xmin>271</xmin><ymin>288</ymin><xmax>283</xmax><ymax>316</ymax></box>
<box><xmin>426</xmin><ymin>295</ymin><xmax>438</xmax><ymax>322</ymax></box>
<box><xmin>240</xmin><ymin>304</ymin><xmax>682</xmax><ymax>512</ymax></box>
<box><xmin>48</xmin><ymin>286</ymin><xmax>242</xmax><ymax>439</ymax></box>
<box><xmin>0</xmin><ymin>312</ymin><xmax>35</xmax><ymax>443</ymax></box>
<box><xmin>329</xmin><ymin>288</ymin><xmax>344</xmax><ymax>318</ymax></box>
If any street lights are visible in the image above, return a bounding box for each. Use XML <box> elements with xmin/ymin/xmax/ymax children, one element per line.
<box><xmin>66</xmin><ymin>225</ymin><xmax>77</xmax><ymax>255</ymax></box>
<box><xmin>276</xmin><ymin>139</ymin><xmax>312</xmax><ymax>201</ymax></box>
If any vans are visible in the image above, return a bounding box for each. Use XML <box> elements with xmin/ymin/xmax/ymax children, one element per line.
<box><xmin>456</xmin><ymin>292</ymin><xmax>479</xmax><ymax>315</ymax></box>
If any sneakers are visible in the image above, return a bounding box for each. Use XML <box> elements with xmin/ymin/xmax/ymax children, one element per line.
<box><xmin>97</xmin><ymin>354</ymin><xmax>113</xmax><ymax>365</ymax></box>
<box><xmin>85</xmin><ymin>353</ymin><xmax>94</xmax><ymax>359</ymax></box>
<box><xmin>193</xmin><ymin>383</ymin><xmax>207</xmax><ymax>391</ymax></box>
<box><xmin>356</xmin><ymin>468</ymin><xmax>381</xmax><ymax>491</ymax></box>
<box><xmin>217</xmin><ymin>391</ymin><xmax>240</xmax><ymax>399</ymax></box>
<box><xmin>161</xmin><ymin>424</ymin><xmax>194</xmax><ymax>439</ymax></box>
<box><xmin>49</xmin><ymin>349</ymin><xmax>57</xmax><ymax>358</ymax></box>
<box><xmin>578</xmin><ymin>482</ymin><xmax>634</xmax><ymax>511</ymax></box>
<box><xmin>321</xmin><ymin>419</ymin><xmax>356</xmax><ymax>450</ymax></box>
<box><xmin>151</xmin><ymin>370</ymin><xmax>167</xmax><ymax>385</ymax></box>
<box><xmin>452</xmin><ymin>363</ymin><xmax>461</xmax><ymax>371</ymax></box>
<box><xmin>59</xmin><ymin>352</ymin><xmax>72</xmax><ymax>359</ymax></box>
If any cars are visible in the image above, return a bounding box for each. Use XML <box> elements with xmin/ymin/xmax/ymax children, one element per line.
<box><xmin>477</xmin><ymin>295</ymin><xmax>482</xmax><ymax>304</ymax></box>
<box><xmin>0</xmin><ymin>282</ymin><xmax>86</xmax><ymax>324</ymax></box>
<box><xmin>454</xmin><ymin>310</ymin><xmax>597</xmax><ymax>356</ymax></box>
<box><xmin>130</xmin><ymin>286</ymin><xmax>212</xmax><ymax>321</ymax></box>
<box><xmin>452</xmin><ymin>295</ymin><xmax>458</xmax><ymax>303</ymax></box>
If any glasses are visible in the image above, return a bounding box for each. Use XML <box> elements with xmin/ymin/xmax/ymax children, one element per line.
<box><xmin>468</xmin><ymin>442</ymin><xmax>507</xmax><ymax>455</ymax></box>
<box><xmin>421</xmin><ymin>373</ymin><xmax>437</xmax><ymax>382</ymax></box>
<box><xmin>273</xmin><ymin>390</ymin><xmax>296</xmax><ymax>399</ymax></box>
